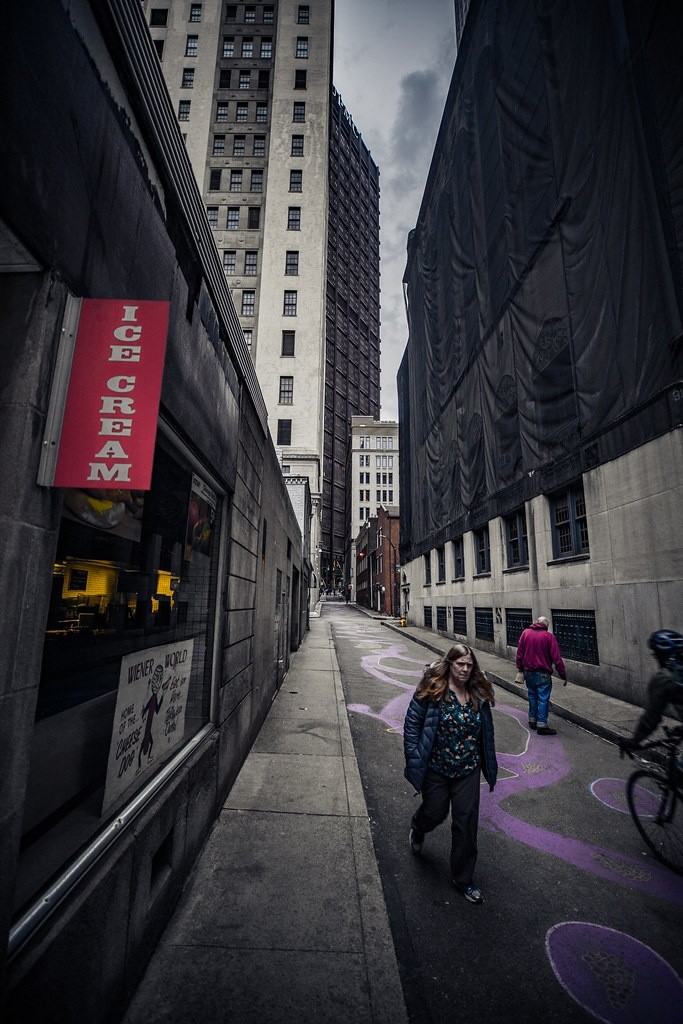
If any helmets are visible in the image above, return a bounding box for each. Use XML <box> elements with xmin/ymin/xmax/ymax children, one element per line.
<box><xmin>648</xmin><ymin>629</ymin><xmax>683</xmax><ymax>656</ymax></box>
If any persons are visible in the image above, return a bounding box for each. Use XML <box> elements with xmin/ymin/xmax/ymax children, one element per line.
<box><xmin>516</xmin><ymin>616</ymin><xmax>567</xmax><ymax>735</ymax></box>
<box><xmin>621</xmin><ymin>630</ymin><xmax>683</xmax><ymax>756</ymax></box>
<box><xmin>403</xmin><ymin>644</ymin><xmax>498</xmax><ymax>904</ymax></box>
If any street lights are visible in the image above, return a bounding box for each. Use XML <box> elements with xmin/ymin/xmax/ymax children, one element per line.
<box><xmin>379</xmin><ymin>535</ymin><xmax>397</xmax><ymax>617</ymax></box>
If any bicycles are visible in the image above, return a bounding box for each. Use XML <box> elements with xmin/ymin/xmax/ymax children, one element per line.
<box><xmin>617</xmin><ymin>725</ymin><xmax>683</xmax><ymax>876</ymax></box>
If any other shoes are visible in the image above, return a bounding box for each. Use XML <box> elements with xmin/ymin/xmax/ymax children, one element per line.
<box><xmin>537</xmin><ymin>727</ymin><xmax>557</xmax><ymax>736</ymax></box>
<box><xmin>529</xmin><ymin>722</ymin><xmax>537</xmax><ymax>729</ymax></box>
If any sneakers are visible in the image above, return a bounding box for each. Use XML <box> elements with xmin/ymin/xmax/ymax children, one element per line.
<box><xmin>451</xmin><ymin>878</ymin><xmax>484</xmax><ymax>905</ymax></box>
<box><xmin>408</xmin><ymin>824</ymin><xmax>425</xmax><ymax>853</ymax></box>
